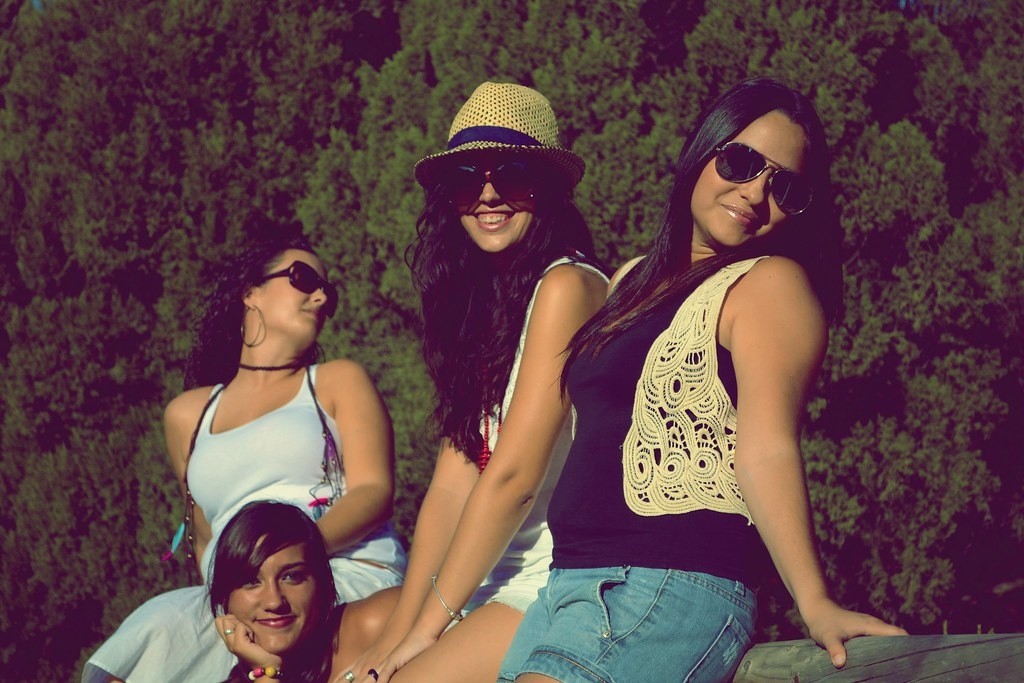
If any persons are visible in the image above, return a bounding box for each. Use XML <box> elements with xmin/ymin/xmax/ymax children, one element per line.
<box><xmin>80</xmin><ymin>236</ymin><xmax>405</xmax><ymax>683</ymax></box>
<box><xmin>333</xmin><ymin>74</ymin><xmax>611</xmax><ymax>683</ymax></box>
<box><xmin>494</xmin><ymin>75</ymin><xmax>910</xmax><ymax>683</ymax></box>
<box><xmin>210</xmin><ymin>498</ymin><xmax>402</xmax><ymax>683</ymax></box>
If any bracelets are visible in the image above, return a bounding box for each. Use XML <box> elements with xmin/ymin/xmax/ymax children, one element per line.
<box><xmin>242</xmin><ymin>666</ymin><xmax>285</xmax><ymax>683</ymax></box>
<box><xmin>430</xmin><ymin>575</ymin><xmax>464</xmax><ymax>622</ymax></box>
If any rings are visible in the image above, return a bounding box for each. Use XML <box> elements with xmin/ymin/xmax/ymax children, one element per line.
<box><xmin>368</xmin><ymin>668</ymin><xmax>379</xmax><ymax>681</ymax></box>
<box><xmin>344</xmin><ymin>671</ymin><xmax>355</xmax><ymax>682</ymax></box>
<box><xmin>223</xmin><ymin>630</ymin><xmax>234</xmax><ymax>635</ymax></box>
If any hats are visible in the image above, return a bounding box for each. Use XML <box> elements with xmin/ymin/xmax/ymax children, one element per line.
<box><xmin>414</xmin><ymin>82</ymin><xmax>586</xmax><ymax>195</ymax></box>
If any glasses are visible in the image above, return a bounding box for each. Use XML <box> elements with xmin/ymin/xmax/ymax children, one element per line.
<box><xmin>715</xmin><ymin>142</ymin><xmax>816</xmax><ymax>216</ymax></box>
<box><xmin>254</xmin><ymin>260</ymin><xmax>338</xmax><ymax>320</ymax></box>
<box><xmin>442</xmin><ymin>157</ymin><xmax>544</xmax><ymax>207</ymax></box>
